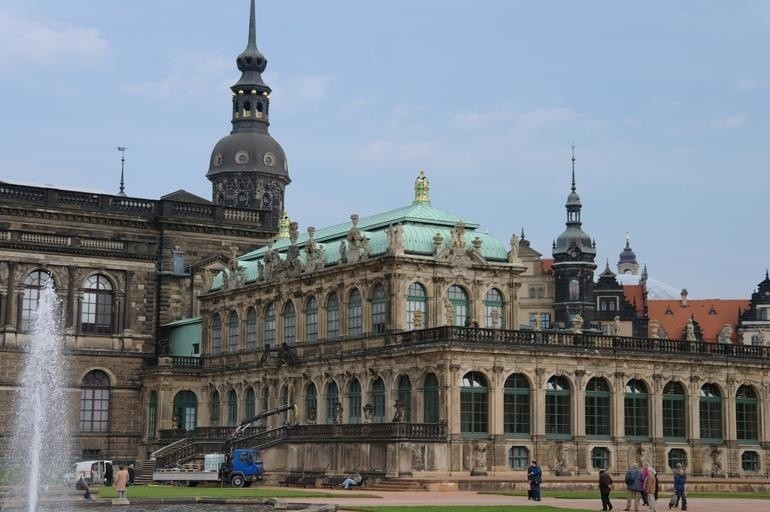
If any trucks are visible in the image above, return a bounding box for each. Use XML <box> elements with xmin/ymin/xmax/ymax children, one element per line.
<box><xmin>61</xmin><ymin>458</ymin><xmax>114</xmax><ymax>488</ymax></box>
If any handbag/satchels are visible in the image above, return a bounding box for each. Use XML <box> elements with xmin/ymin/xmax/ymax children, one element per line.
<box><xmin>533</xmin><ymin>476</ymin><xmax>542</xmax><ymax>483</ymax></box>
<box><xmin>670</xmin><ymin>495</ymin><xmax>678</xmax><ymax>504</ymax></box>
<box><xmin>628</xmin><ymin>478</ymin><xmax>635</xmax><ymax>484</ymax></box>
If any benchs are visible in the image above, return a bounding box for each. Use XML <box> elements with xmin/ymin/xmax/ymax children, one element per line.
<box><xmin>278</xmin><ymin>475</ymin><xmax>316</xmax><ymax>488</ymax></box>
<box><xmin>320</xmin><ymin>475</ymin><xmax>369</xmax><ymax>490</ymax></box>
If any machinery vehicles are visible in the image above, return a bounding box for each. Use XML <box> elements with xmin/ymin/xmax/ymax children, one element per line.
<box><xmin>152</xmin><ymin>404</ymin><xmax>301</xmax><ymax>489</ymax></box>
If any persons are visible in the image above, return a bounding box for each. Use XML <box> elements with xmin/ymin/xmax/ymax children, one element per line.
<box><xmin>625</xmin><ymin>465</ymin><xmax>632</xmax><ymax>484</ymax></box>
<box><xmin>598</xmin><ymin>466</ymin><xmax>615</xmax><ymax>511</ymax></box>
<box><xmin>337</xmin><ymin>471</ymin><xmax>363</xmax><ymax>489</ymax></box>
<box><xmin>106</xmin><ymin>460</ymin><xmax>114</xmax><ymax>486</ymax></box>
<box><xmin>668</xmin><ymin>462</ymin><xmax>687</xmax><ymax>510</ymax></box>
<box><xmin>530</xmin><ymin>458</ymin><xmax>542</xmax><ymax>501</ymax></box>
<box><xmin>76</xmin><ymin>475</ymin><xmax>91</xmax><ymax>498</ymax></box>
<box><xmin>645</xmin><ymin>466</ymin><xmax>658</xmax><ymax>512</ymax></box>
<box><xmin>114</xmin><ymin>464</ymin><xmax>130</xmax><ymax>498</ymax></box>
<box><xmin>128</xmin><ymin>463</ymin><xmax>135</xmax><ymax>486</ymax></box>
<box><xmin>528</xmin><ymin>464</ymin><xmax>543</xmax><ymax>500</ymax></box>
<box><xmin>639</xmin><ymin>461</ymin><xmax>650</xmax><ymax>506</ymax></box>
<box><xmin>624</xmin><ymin>463</ymin><xmax>643</xmax><ymax>511</ymax></box>
